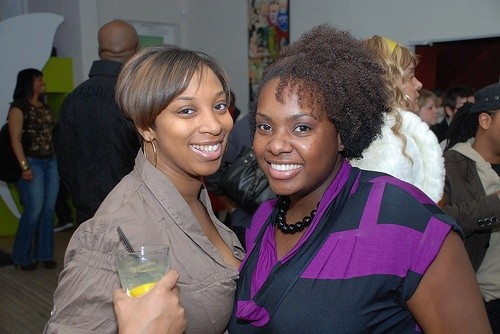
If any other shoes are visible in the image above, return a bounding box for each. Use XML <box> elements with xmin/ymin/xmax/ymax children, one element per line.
<box><xmin>16</xmin><ymin>262</ymin><xmax>35</xmax><ymax>270</ymax></box>
<box><xmin>35</xmin><ymin>259</ymin><xmax>57</xmax><ymax>269</ymax></box>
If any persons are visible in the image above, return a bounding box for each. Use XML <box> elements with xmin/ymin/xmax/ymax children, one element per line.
<box><xmin>439</xmin><ymin>84</ymin><xmax>500</xmax><ymax>334</ymax></box>
<box><xmin>349</xmin><ymin>35</ymin><xmax>476</xmax><ymax>205</ymax></box>
<box><xmin>55</xmin><ymin>20</ymin><xmax>144</xmax><ymax>226</ymax></box>
<box><xmin>43</xmin><ymin>46</ymin><xmax>245</xmax><ymax>334</ymax></box>
<box><xmin>227</xmin><ymin>25</ymin><xmax>493</xmax><ymax>333</ymax></box>
<box><xmin>0</xmin><ymin>68</ymin><xmax>75</xmax><ymax>270</ymax></box>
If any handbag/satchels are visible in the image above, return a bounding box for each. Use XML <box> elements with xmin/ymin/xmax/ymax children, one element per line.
<box><xmin>0</xmin><ymin>123</ymin><xmax>33</xmax><ymax>183</ymax></box>
<box><xmin>219</xmin><ymin>144</ymin><xmax>277</xmax><ymax>208</ymax></box>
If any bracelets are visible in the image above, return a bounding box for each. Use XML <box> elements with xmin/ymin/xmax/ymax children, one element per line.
<box><xmin>20</xmin><ymin>161</ymin><xmax>30</xmax><ymax>170</ymax></box>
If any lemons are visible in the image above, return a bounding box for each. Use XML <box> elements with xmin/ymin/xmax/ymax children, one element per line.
<box><xmin>129</xmin><ymin>282</ymin><xmax>157</xmax><ymax>297</ymax></box>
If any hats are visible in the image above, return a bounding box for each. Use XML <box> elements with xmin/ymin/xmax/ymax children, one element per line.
<box><xmin>470</xmin><ymin>82</ymin><xmax>500</xmax><ymax>113</ymax></box>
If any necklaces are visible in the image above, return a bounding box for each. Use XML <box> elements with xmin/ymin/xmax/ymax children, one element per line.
<box><xmin>277</xmin><ymin>197</ymin><xmax>321</xmax><ymax>234</ymax></box>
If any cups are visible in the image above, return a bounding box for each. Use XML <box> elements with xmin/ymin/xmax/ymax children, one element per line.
<box><xmin>114</xmin><ymin>245</ymin><xmax>170</xmax><ymax>298</ymax></box>
